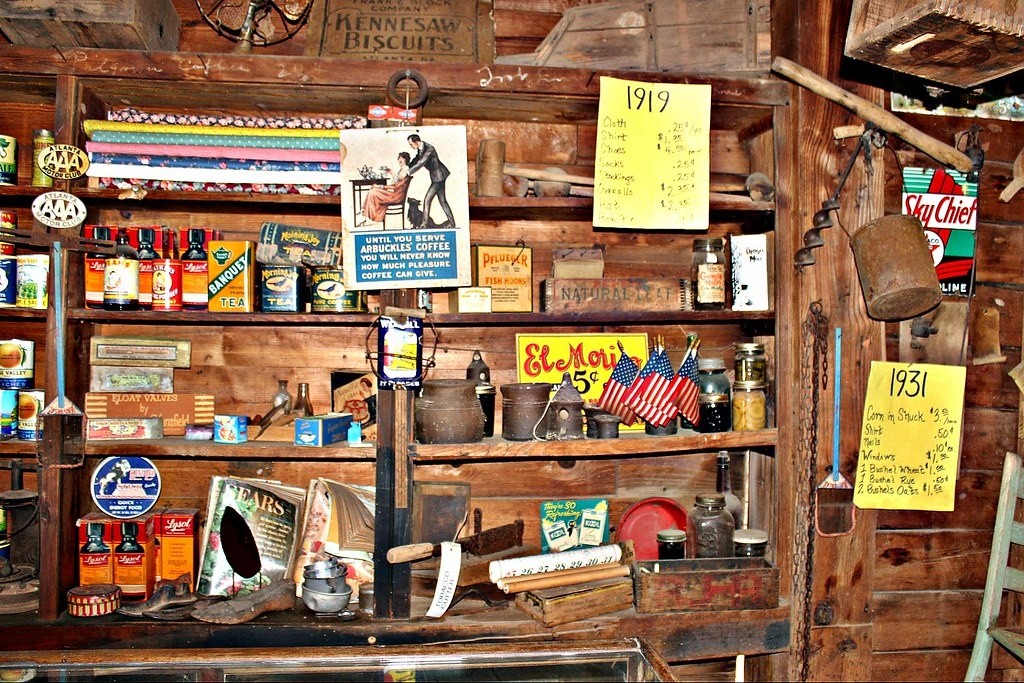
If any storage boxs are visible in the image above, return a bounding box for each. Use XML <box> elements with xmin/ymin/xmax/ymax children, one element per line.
<box><xmin>293</xmin><ymin>367</ymin><xmax>378</xmax><ymax>447</ymax></box>
<box><xmin>84</xmin><ymin>222</ymin><xmax>371</xmax><ymax>312</ymax></box>
<box><xmin>86</xmin><ymin>333</ymin><xmax>214</xmax><ymax>439</ymax></box>
<box><xmin>515</xmin><ymin>576</ymin><xmax>632</xmax><ymax>626</ymax></box>
<box><xmin>79</xmin><ymin>501</ymin><xmax>199</xmax><ymax>603</ymax></box>
<box><xmin>630</xmin><ymin>556</ymin><xmax>780</xmax><ymax>612</ymax></box>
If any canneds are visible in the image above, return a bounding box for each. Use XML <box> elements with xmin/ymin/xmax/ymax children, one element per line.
<box><xmin>150</xmin><ymin>257</ymin><xmax>184</xmax><ymax>314</ymax></box>
<box><xmin>0</xmin><ymin>210</ymin><xmax>50</xmax><ymax>309</ymax></box>
<box><xmin>732</xmin><ymin>529</ymin><xmax>770</xmax><ymax>559</ymax></box>
<box><xmin>691</xmin><ymin>238</ymin><xmax>729</xmax><ymax>312</ymax></box>
<box><xmin>0</xmin><ymin>337</ymin><xmax>46</xmax><ymax>442</ymax></box>
<box><xmin>656</xmin><ymin>528</ymin><xmax>688</xmax><ymax>561</ymax></box>
<box><xmin>0</xmin><ymin>135</ymin><xmax>18</xmax><ymax>188</ymax></box>
<box><xmin>31</xmin><ymin>128</ymin><xmax>54</xmax><ymax>187</ymax></box>
<box><xmin>697</xmin><ymin>342</ymin><xmax>770</xmax><ymax>434</ymax></box>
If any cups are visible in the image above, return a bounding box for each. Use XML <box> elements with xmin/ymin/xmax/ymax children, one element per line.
<box><xmin>501</xmin><ymin>382</ymin><xmax>552</xmax><ymax>440</ymax></box>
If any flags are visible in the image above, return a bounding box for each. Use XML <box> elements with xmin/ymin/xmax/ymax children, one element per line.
<box><xmin>597</xmin><ymin>348</ymin><xmax>700</xmax><ymax>427</ymax></box>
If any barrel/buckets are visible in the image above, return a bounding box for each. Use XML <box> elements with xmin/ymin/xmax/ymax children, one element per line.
<box><xmin>0</xmin><ymin>338</ymin><xmax>45</xmax><ymax>441</ymax></box>
<box><xmin>818</xmin><ymin>131</ymin><xmax>942</xmax><ymax>322</ymax></box>
<box><xmin>0</xmin><ymin>128</ymin><xmax>54</xmax><ymax>311</ymax></box>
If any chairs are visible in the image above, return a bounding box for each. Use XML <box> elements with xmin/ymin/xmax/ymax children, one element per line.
<box><xmin>965</xmin><ymin>451</ymin><xmax>1024</xmax><ymax>683</ymax></box>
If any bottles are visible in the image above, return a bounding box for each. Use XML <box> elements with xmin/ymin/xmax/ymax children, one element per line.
<box><xmin>81</xmin><ymin>523</ymin><xmax>110</xmax><ymax>553</ymax></box>
<box><xmin>115</xmin><ymin>522</ymin><xmax>145</xmax><ymax>600</ymax></box>
<box><xmin>692</xmin><ymin>340</ymin><xmax>768</xmax><ymax>434</ymax></box>
<box><xmin>688</xmin><ymin>236</ymin><xmax>725</xmax><ymax>311</ymax></box>
<box><xmin>415</xmin><ymin>378</ymin><xmax>483</xmax><ymax>443</ymax></box>
<box><xmin>103</xmin><ymin>233</ymin><xmax>139</xmax><ymax>309</ymax></box>
<box><xmin>655</xmin><ymin>451</ymin><xmax>769</xmax><ymax>559</ymax></box>
<box><xmin>84</xmin><ymin>223</ymin><xmax>212</xmax><ymax>312</ymax></box>
<box><xmin>475</xmin><ymin>384</ymin><xmax>496</xmax><ymax>436</ymax></box>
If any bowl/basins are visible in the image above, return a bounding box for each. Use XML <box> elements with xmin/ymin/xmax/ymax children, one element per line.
<box><xmin>615</xmin><ymin>497</ymin><xmax>689</xmax><ymax>560</ymax></box>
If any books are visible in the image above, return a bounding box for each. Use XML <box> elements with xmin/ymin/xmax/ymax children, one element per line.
<box><xmin>196</xmin><ymin>475</ymin><xmax>376</xmax><ymax>603</ymax></box>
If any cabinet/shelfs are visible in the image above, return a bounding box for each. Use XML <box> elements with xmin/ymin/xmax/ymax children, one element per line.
<box><xmin>59</xmin><ymin>47</ymin><xmax>392</xmax><ymax>646</ymax></box>
<box><xmin>384</xmin><ymin>53</ymin><xmax>793</xmax><ymax>662</ymax></box>
<box><xmin>0</xmin><ymin>41</ymin><xmax>74</xmax><ymax>650</ymax></box>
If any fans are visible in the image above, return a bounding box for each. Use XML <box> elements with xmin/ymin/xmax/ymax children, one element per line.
<box><xmin>195</xmin><ymin>0</ymin><xmax>314</xmax><ymax>57</ymax></box>
